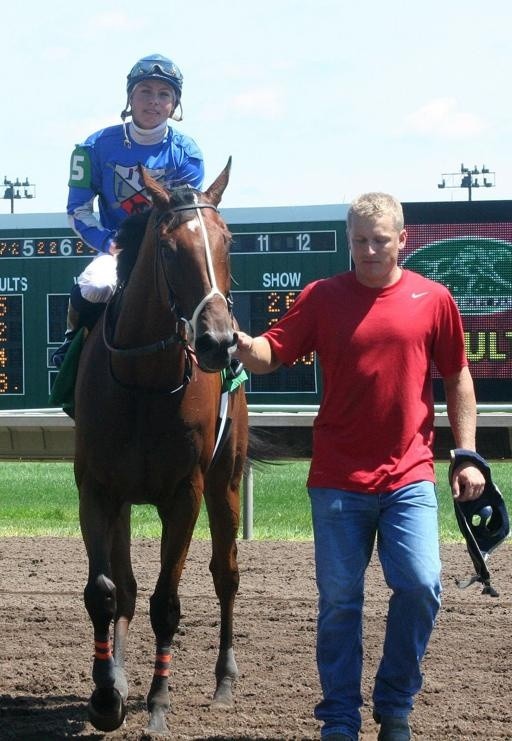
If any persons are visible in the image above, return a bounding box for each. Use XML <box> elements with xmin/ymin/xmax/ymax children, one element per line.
<box><xmin>234</xmin><ymin>190</ymin><xmax>486</xmax><ymax>740</ymax></box>
<box><xmin>46</xmin><ymin>53</ymin><xmax>248</xmax><ymax>382</ymax></box>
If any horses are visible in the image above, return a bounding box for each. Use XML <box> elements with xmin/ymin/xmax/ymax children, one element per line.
<box><xmin>70</xmin><ymin>153</ymin><xmax>292</xmax><ymax>736</ymax></box>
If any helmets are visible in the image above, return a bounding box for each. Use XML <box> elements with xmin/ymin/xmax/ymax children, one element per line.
<box><xmin>127</xmin><ymin>54</ymin><xmax>184</xmax><ymax>106</ymax></box>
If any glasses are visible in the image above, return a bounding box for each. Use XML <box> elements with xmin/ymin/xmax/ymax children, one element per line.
<box><xmin>130</xmin><ymin>61</ymin><xmax>181</xmax><ymax>79</ymax></box>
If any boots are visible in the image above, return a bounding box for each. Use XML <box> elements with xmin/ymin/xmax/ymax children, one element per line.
<box><xmin>53</xmin><ymin>297</ymin><xmax>89</xmax><ymax>371</ymax></box>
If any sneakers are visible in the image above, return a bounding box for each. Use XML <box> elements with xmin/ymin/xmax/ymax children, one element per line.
<box><xmin>375</xmin><ymin>716</ymin><xmax>412</xmax><ymax>741</ymax></box>
